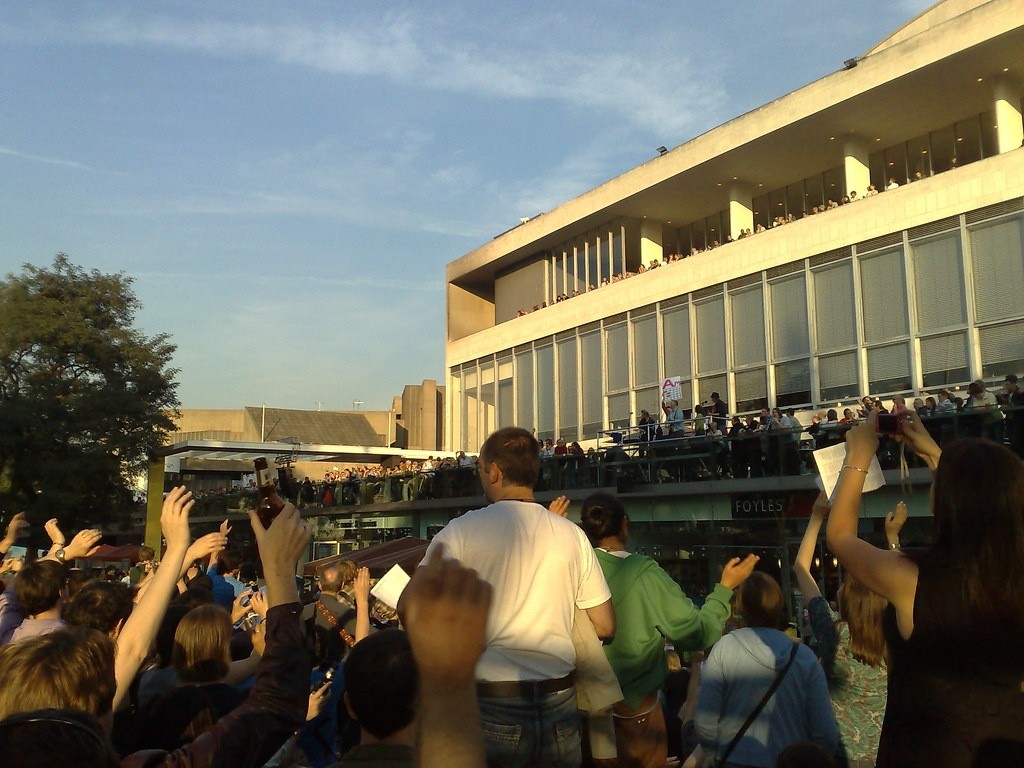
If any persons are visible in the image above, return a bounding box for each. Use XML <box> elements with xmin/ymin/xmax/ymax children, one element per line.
<box><xmin>515</xmin><ymin>154</ymin><xmax>957</xmax><ymax>320</ymax></box>
<box><xmin>0</xmin><ymin>371</ymin><xmax>1024</xmax><ymax>768</ymax></box>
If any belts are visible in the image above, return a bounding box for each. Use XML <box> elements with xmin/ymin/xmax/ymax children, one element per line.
<box><xmin>476</xmin><ymin>671</ymin><xmax>573</xmax><ymax>697</ymax></box>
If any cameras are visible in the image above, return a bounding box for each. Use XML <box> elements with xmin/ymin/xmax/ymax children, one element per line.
<box><xmin>875</xmin><ymin>414</ymin><xmax>903</xmax><ymax>435</ymax></box>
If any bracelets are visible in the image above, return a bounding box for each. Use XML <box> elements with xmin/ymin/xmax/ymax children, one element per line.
<box><xmin>841</xmin><ymin>463</ymin><xmax>871</xmax><ymax>476</ymax></box>
<box><xmin>51</xmin><ymin>541</ymin><xmax>65</xmax><ymax>548</ymax></box>
<box><xmin>887</xmin><ymin>543</ymin><xmax>901</xmax><ymax>549</ymax></box>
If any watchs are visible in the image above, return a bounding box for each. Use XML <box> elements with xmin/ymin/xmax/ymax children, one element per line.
<box><xmin>55</xmin><ymin>548</ymin><xmax>67</xmax><ymax>564</ymax></box>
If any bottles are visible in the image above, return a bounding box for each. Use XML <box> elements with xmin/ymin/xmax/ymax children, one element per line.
<box><xmin>253</xmin><ymin>457</ymin><xmax>298</xmax><ymax>575</ymax></box>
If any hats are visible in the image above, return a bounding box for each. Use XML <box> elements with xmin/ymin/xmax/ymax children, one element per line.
<box><xmin>711</xmin><ymin>393</ymin><xmax>719</xmax><ymax>398</ymax></box>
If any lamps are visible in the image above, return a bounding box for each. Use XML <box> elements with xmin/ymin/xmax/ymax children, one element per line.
<box><xmin>844</xmin><ymin>58</ymin><xmax>857</xmax><ymax>69</ymax></box>
<box><xmin>657</xmin><ymin>146</ymin><xmax>668</xmax><ymax>155</ymax></box>
